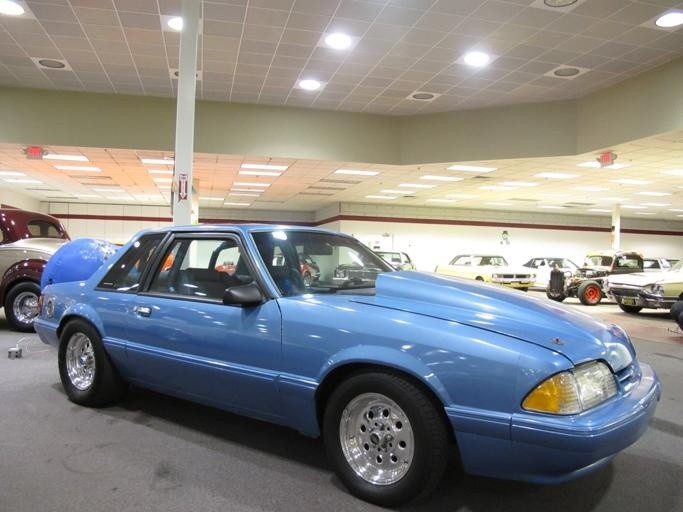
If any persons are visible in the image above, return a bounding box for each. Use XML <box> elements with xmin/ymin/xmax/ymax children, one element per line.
<box><xmin>498</xmin><ymin>230</ymin><xmax>513</xmax><ymax>245</ymax></box>
<box><xmin>215</xmin><ymin>262</ymin><xmax>237</xmax><ymax>273</ymax></box>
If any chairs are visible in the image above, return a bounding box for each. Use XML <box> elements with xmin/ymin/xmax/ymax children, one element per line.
<box><xmin>180</xmin><ymin>268</ymin><xmax>234</xmax><ymax>301</ymax></box>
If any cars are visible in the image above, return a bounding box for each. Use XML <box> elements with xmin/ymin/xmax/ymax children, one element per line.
<box><xmin>602</xmin><ymin>257</ymin><xmax>682</xmax><ymax>325</ymax></box>
<box><xmin>35</xmin><ymin>221</ymin><xmax>664</xmax><ymax>504</ymax></box>
<box><xmin>0</xmin><ymin>202</ymin><xmax>70</xmax><ymax>337</ymax></box>
